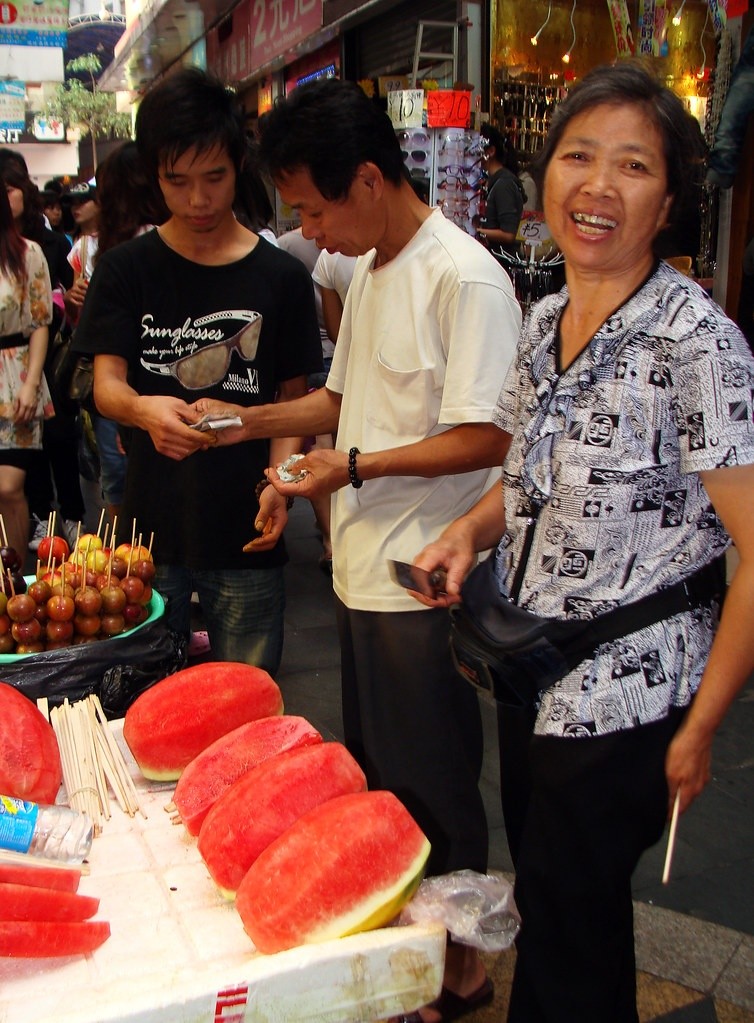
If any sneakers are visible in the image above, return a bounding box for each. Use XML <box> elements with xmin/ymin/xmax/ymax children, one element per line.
<box><xmin>61</xmin><ymin>519</ymin><xmax>86</xmax><ymax>551</ymax></box>
<box><xmin>28</xmin><ymin>513</ymin><xmax>50</xmax><ymax>550</ymax></box>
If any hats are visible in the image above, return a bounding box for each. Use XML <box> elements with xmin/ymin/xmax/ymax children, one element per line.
<box><xmin>67</xmin><ymin>182</ymin><xmax>96</xmax><ymax>200</ymax></box>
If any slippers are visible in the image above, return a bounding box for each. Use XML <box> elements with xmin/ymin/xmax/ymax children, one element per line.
<box><xmin>398</xmin><ymin>974</ymin><xmax>494</xmax><ymax>1023</ymax></box>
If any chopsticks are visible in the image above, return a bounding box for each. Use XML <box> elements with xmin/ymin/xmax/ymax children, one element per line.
<box><xmin>50</xmin><ymin>693</ymin><xmax>148</xmax><ymax>841</ymax></box>
<box><xmin>663</xmin><ymin>788</ymin><xmax>686</xmax><ymax>885</ymax></box>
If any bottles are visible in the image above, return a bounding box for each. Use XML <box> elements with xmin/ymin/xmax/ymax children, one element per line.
<box><xmin>0</xmin><ymin>794</ymin><xmax>95</xmax><ymax>864</ymax></box>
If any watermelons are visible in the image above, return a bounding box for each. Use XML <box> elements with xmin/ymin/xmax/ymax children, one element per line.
<box><xmin>122</xmin><ymin>661</ymin><xmax>432</xmax><ymax>959</ymax></box>
<box><xmin>0</xmin><ymin>859</ymin><xmax>111</xmax><ymax>958</ymax></box>
<box><xmin>0</xmin><ymin>681</ymin><xmax>62</xmax><ymax>806</ymax></box>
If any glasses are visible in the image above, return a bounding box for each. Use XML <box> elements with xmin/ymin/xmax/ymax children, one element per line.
<box><xmin>401</xmin><ymin>150</ymin><xmax>428</xmax><ymax>163</ymax></box>
<box><xmin>397</xmin><ymin>132</ymin><xmax>428</xmax><ymax>147</ymax></box>
<box><xmin>408</xmin><ymin>168</ymin><xmax>427</xmax><ymax>177</ymax></box>
<box><xmin>437</xmin><ymin>133</ymin><xmax>489</xmax><ymax>244</ymax></box>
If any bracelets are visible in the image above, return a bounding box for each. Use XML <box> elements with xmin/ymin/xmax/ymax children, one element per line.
<box><xmin>348</xmin><ymin>446</ymin><xmax>363</xmax><ymax>490</ymax></box>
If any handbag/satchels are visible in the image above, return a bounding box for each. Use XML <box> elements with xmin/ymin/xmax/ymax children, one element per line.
<box><xmin>448</xmin><ymin>553</ymin><xmax>726</xmax><ymax>705</ymax></box>
<box><xmin>44</xmin><ymin>330</ymin><xmax>94</xmax><ymax>404</ymax></box>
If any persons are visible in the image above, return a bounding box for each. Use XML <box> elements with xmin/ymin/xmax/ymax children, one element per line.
<box><xmin>0</xmin><ymin>63</ymin><xmax>754</xmax><ymax>1023</ymax></box>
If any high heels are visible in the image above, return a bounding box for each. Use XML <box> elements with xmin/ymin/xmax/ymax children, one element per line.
<box><xmin>318</xmin><ymin>555</ymin><xmax>332</xmax><ymax>571</ymax></box>
